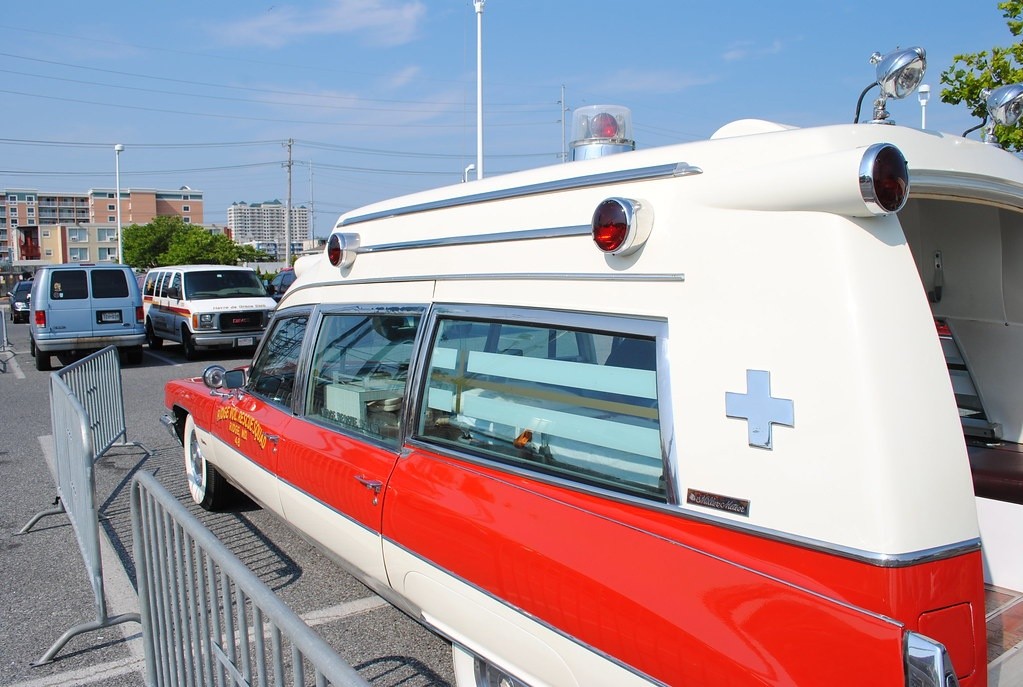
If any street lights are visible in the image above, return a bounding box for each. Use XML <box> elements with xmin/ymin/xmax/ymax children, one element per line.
<box><xmin>473</xmin><ymin>0</ymin><xmax>486</xmax><ymax>179</ymax></box>
<box><xmin>916</xmin><ymin>84</ymin><xmax>930</xmax><ymax>130</ymax></box>
<box><xmin>114</xmin><ymin>144</ymin><xmax>125</xmax><ymax>266</ymax></box>
<box><xmin>465</xmin><ymin>163</ymin><xmax>476</xmax><ymax>182</ymax></box>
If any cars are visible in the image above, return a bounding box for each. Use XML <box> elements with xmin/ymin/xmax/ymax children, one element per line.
<box><xmin>266</xmin><ymin>270</ymin><xmax>296</xmax><ymax>304</ymax></box>
<box><xmin>7</xmin><ymin>280</ymin><xmax>33</xmax><ymax>324</ymax></box>
<box><xmin>130</xmin><ymin>268</ymin><xmax>148</xmax><ymax>294</ymax></box>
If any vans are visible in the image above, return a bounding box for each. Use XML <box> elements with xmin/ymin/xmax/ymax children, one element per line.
<box><xmin>14</xmin><ymin>261</ymin><xmax>146</xmax><ymax>371</ymax></box>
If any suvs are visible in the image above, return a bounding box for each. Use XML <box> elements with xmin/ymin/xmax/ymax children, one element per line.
<box><xmin>142</xmin><ymin>265</ymin><xmax>280</xmax><ymax>362</ymax></box>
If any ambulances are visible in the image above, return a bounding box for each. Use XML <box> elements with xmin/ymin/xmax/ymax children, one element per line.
<box><xmin>159</xmin><ymin>44</ymin><xmax>1023</xmax><ymax>687</ymax></box>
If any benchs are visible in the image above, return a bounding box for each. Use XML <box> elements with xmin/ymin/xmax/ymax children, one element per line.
<box><xmin>964</xmin><ymin>434</ymin><xmax>1023</xmax><ymax>505</ymax></box>
